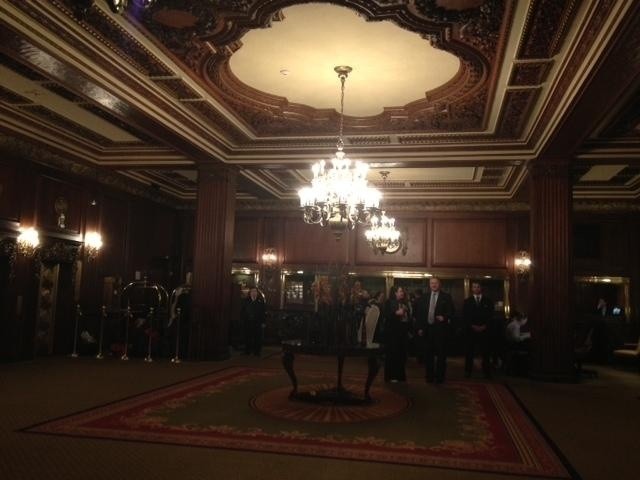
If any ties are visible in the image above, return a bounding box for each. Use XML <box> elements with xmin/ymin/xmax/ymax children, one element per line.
<box><xmin>477</xmin><ymin>296</ymin><xmax>480</xmax><ymax>307</ymax></box>
<box><xmin>429</xmin><ymin>292</ymin><xmax>436</xmax><ymax>323</ymax></box>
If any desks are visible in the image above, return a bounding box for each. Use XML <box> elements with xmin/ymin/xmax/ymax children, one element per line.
<box><xmin>281</xmin><ymin>338</ymin><xmax>385</xmax><ymax>401</ymax></box>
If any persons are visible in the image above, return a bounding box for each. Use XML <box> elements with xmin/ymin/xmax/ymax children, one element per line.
<box><xmin>240</xmin><ymin>287</ymin><xmax>268</xmax><ymax>357</ymax></box>
<box><xmin>460</xmin><ymin>281</ymin><xmax>495</xmax><ymax>380</ymax></box>
<box><xmin>381</xmin><ymin>284</ymin><xmax>409</xmax><ymax>383</ymax></box>
<box><xmin>412</xmin><ymin>288</ymin><xmax>423</xmax><ymax>367</ymax></box>
<box><xmin>415</xmin><ymin>276</ymin><xmax>458</xmax><ymax>384</ymax></box>
<box><xmin>369</xmin><ymin>292</ymin><xmax>385</xmax><ymax>311</ymax></box>
<box><xmin>505</xmin><ymin>312</ymin><xmax>538</xmax><ymax>365</ymax></box>
<box><xmin>591</xmin><ymin>295</ymin><xmax>616</xmax><ymax>369</ymax></box>
<box><xmin>504</xmin><ymin>312</ymin><xmax>518</xmax><ymax>331</ymax></box>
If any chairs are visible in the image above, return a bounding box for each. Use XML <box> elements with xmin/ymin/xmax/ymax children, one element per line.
<box><xmin>120</xmin><ymin>280</ymin><xmax>192</xmax><ymax>358</ymax></box>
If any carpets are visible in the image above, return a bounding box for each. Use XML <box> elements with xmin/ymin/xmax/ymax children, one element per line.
<box><xmin>15</xmin><ymin>349</ymin><xmax>582</xmax><ymax>479</ymax></box>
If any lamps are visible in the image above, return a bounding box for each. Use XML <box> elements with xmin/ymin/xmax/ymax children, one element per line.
<box><xmin>262</xmin><ymin>247</ymin><xmax>277</xmax><ymax>266</ymax></box>
<box><xmin>514</xmin><ymin>250</ymin><xmax>532</xmax><ymax>281</ymax></box>
<box><xmin>295</xmin><ymin>66</ymin><xmax>409</xmax><ymax>256</ymax></box>
